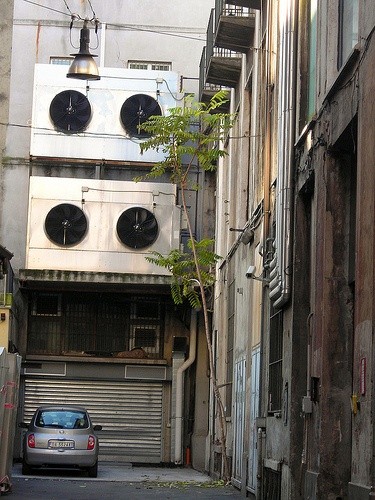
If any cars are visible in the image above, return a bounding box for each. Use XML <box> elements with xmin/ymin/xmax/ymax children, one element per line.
<box><xmin>18</xmin><ymin>405</ymin><xmax>102</xmax><ymax>478</ymax></box>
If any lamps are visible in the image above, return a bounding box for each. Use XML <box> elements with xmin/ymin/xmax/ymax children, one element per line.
<box><xmin>67</xmin><ymin>25</ymin><xmax>102</xmax><ymax>82</ymax></box>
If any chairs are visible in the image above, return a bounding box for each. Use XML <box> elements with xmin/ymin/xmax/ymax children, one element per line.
<box><xmin>41</xmin><ymin>414</ymin><xmax>54</xmax><ymax>427</ymax></box>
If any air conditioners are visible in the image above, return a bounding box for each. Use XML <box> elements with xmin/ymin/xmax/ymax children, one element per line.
<box><xmin>31</xmin><ymin>63</ymin><xmax>181</xmax><ymax>165</ymax></box>
<box><xmin>25</xmin><ymin>178</ymin><xmax>180</xmax><ymax>279</ymax></box>
